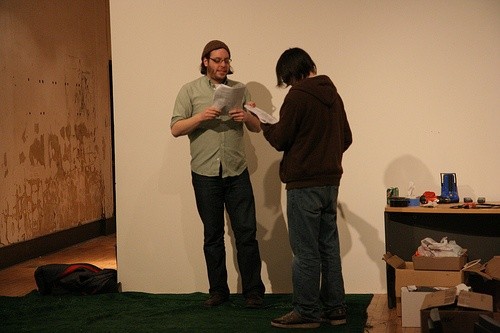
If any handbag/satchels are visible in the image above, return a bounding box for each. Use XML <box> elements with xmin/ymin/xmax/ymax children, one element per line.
<box><xmin>34</xmin><ymin>262</ymin><xmax>118</xmax><ymax>294</ymax></box>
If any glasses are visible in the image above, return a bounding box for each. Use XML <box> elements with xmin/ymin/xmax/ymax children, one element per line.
<box><xmin>208</xmin><ymin>58</ymin><xmax>232</xmax><ymax>64</ymax></box>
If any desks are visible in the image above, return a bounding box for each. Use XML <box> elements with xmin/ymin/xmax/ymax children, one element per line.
<box><xmin>383</xmin><ymin>202</ymin><xmax>500</xmax><ymax>310</ymax></box>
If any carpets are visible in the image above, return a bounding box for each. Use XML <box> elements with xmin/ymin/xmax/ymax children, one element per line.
<box><xmin>0</xmin><ymin>289</ymin><xmax>374</xmax><ymax>333</ymax></box>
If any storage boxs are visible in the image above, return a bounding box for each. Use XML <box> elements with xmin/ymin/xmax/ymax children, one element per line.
<box><xmin>410</xmin><ymin>199</ymin><xmax>422</xmax><ymax>205</ymax></box>
<box><xmin>381</xmin><ymin>249</ymin><xmax>500</xmax><ymax>333</ymax></box>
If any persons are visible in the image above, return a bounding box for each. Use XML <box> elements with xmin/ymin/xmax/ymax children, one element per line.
<box><xmin>246</xmin><ymin>48</ymin><xmax>352</xmax><ymax>328</ymax></box>
<box><xmin>170</xmin><ymin>40</ymin><xmax>265</xmax><ymax>306</ymax></box>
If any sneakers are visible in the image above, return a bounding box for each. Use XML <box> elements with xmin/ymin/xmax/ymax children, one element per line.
<box><xmin>270</xmin><ymin>309</ymin><xmax>321</xmax><ymax>328</ymax></box>
<box><xmin>243</xmin><ymin>292</ymin><xmax>264</xmax><ymax>308</ymax></box>
<box><xmin>205</xmin><ymin>296</ymin><xmax>229</xmax><ymax>305</ymax></box>
<box><xmin>321</xmin><ymin>312</ymin><xmax>347</xmax><ymax>325</ymax></box>
